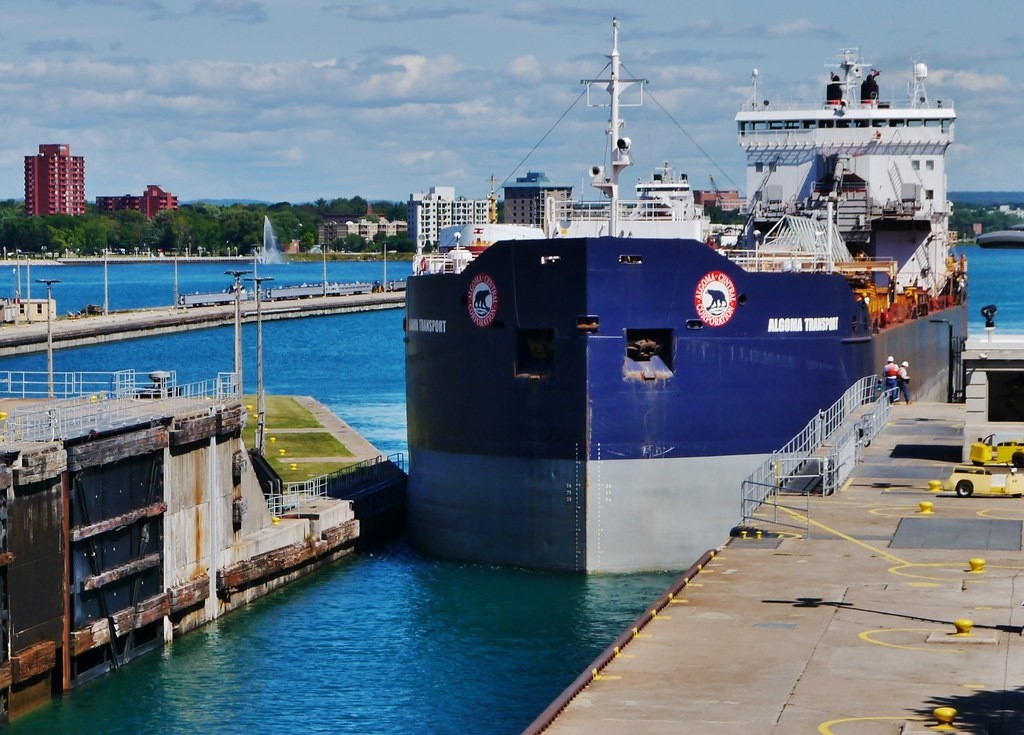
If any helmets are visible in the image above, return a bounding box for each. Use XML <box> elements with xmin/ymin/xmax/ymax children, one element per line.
<box><xmin>901</xmin><ymin>361</ymin><xmax>909</xmax><ymax>368</ymax></box>
<box><xmin>887</xmin><ymin>356</ymin><xmax>894</xmax><ymax>361</ymax></box>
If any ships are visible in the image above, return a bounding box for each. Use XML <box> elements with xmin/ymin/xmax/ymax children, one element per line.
<box><xmin>404</xmin><ymin>16</ymin><xmax>968</xmax><ymax>577</ymax></box>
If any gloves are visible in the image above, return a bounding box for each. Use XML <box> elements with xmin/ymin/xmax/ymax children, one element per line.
<box><xmin>877</xmin><ymin>380</ymin><xmax>882</xmax><ymax>385</ymax></box>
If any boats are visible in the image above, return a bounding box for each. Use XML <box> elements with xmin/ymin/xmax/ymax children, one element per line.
<box><xmin>975</xmin><ymin>221</ymin><xmax>1024</xmax><ymax>249</ymax></box>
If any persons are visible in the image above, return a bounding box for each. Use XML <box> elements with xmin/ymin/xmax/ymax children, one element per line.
<box><xmin>882</xmin><ymin>355</ymin><xmax>899</xmax><ymax>405</ymax></box>
<box><xmin>895</xmin><ymin>361</ymin><xmax>912</xmax><ymax>405</ymax></box>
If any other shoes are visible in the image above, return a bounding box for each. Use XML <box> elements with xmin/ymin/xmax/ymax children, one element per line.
<box><xmin>907</xmin><ymin>402</ymin><xmax>912</xmax><ymax>405</ymax></box>
<box><xmin>893</xmin><ymin>403</ymin><xmax>901</xmax><ymax>406</ymax></box>
